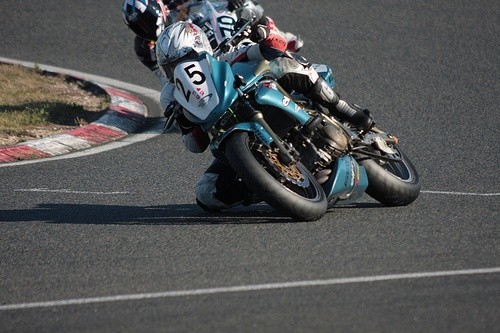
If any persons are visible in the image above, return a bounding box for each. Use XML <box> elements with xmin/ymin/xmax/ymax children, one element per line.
<box><xmin>155</xmin><ymin>23</ymin><xmax>375</xmax><ymax>212</ymax></box>
<box><xmin>121</xmin><ymin>0</ymin><xmax>303</xmax><ymax>90</ymax></box>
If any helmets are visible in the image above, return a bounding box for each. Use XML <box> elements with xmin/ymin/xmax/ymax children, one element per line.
<box><xmin>156</xmin><ymin>21</ymin><xmax>214</xmax><ymax>86</ymax></box>
<box><xmin>121</xmin><ymin>0</ymin><xmax>167</xmax><ymax>43</ymax></box>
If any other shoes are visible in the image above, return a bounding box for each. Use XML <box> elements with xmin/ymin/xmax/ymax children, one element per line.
<box><xmin>303</xmin><ymin>76</ymin><xmax>373</xmax><ymax>131</ymax></box>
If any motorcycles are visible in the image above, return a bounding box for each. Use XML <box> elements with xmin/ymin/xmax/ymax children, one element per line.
<box><xmin>162</xmin><ymin>18</ymin><xmax>420</xmax><ymax>221</ymax></box>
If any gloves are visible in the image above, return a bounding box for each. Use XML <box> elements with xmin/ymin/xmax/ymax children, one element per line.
<box><xmin>233</xmin><ymin>16</ymin><xmax>252</xmax><ymax>38</ymax></box>
<box><xmin>227</xmin><ymin>0</ymin><xmax>249</xmax><ymax>12</ymax></box>
<box><xmin>163</xmin><ymin>100</ymin><xmax>178</xmax><ymax>117</ymax></box>
